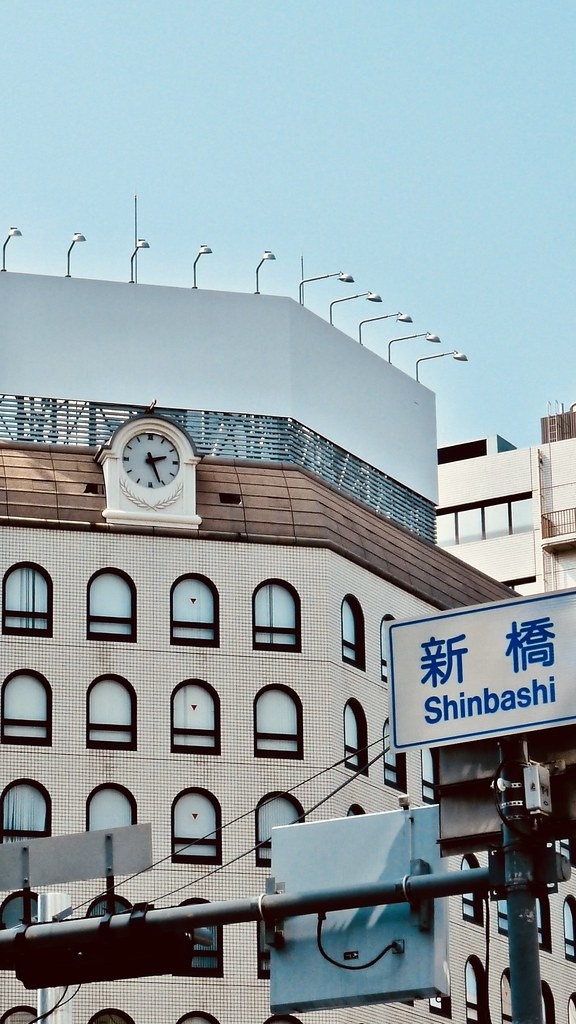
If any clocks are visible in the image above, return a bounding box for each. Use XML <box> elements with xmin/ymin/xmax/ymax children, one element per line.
<box><xmin>122</xmin><ymin>430</ymin><xmax>182</xmax><ymax>491</ymax></box>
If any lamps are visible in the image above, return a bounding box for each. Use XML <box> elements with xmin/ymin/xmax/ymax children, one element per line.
<box><xmin>1</xmin><ymin>225</ymin><xmax>23</xmax><ymax>272</ymax></box>
<box><xmin>358</xmin><ymin>311</ymin><xmax>414</xmax><ymax>345</ymax></box>
<box><xmin>298</xmin><ymin>271</ymin><xmax>355</xmax><ymax>307</ymax></box>
<box><xmin>66</xmin><ymin>232</ymin><xmax>85</xmax><ymax>278</ymax></box>
<box><xmin>329</xmin><ymin>291</ymin><xmax>383</xmax><ymax>326</ymax></box>
<box><xmin>190</xmin><ymin>243</ymin><xmax>213</xmax><ymax>289</ymax></box>
<box><xmin>254</xmin><ymin>250</ymin><xmax>277</xmax><ymax>294</ymax></box>
<box><xmin>128</xmin><ymin>238</ymin><xmax>150</xmax><ymax>283</ymax></box>
<box><xmin>388</xmin><ymin>332</ymin><xmax>442</xmax><ymax>365</ymax></box>
<box><xmin>415</xmin><ymin>349</ymin><xmax>469</xmax><ymax>382</ymax></box>
